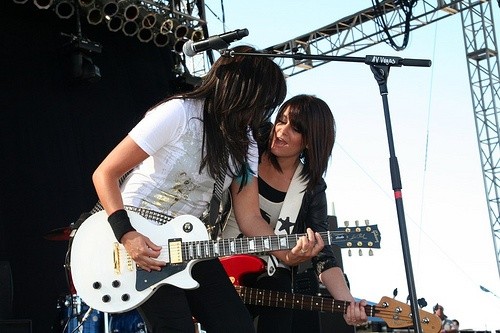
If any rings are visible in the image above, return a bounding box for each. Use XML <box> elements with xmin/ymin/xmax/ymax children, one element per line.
<box><xmin>301</xmin><ymin>249</ymin><xmax>309</xmax><ymax>254</ymax></box>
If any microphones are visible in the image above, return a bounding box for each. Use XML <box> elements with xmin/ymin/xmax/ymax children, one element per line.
<box><xmin>183</xmin><ymin>28</ymin><xmax>249</xmax><ymax>56</ymax></box>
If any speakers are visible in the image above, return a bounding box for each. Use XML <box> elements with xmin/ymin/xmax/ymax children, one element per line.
<box><xmin>0</xmin><ymin>319</ymin><xmax>32</xmax><ymax>333</ymax></box>
<box><xmin>0</xmin><ymin>261</ymin><xmax>14</xmax><ymax>320</ymax></box>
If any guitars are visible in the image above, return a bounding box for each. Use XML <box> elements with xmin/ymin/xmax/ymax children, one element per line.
<box><xmin>217</xmin><ymin>254</ymin><xmax>444</xmax><ymax>333</ymax></box>
<box><xmin>66</xmin><ymin>208</ymin><xmax>382</xmax><ymax>315</ymax></box>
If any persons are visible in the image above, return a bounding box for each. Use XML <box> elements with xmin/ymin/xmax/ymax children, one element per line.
<box><xmin>432</xmin><ymin>304</ymin><xmax>459</xmax><ymax>332</ymax></box>
<box><xmin>91</xmin><ymin>43</ymin><xmax>325</xmax><ymax>333</ymax></box>
<box><xmin>212</xmin><ymin>93</ymin><xmax>371</xmax><ymax>333</ymax></box>
